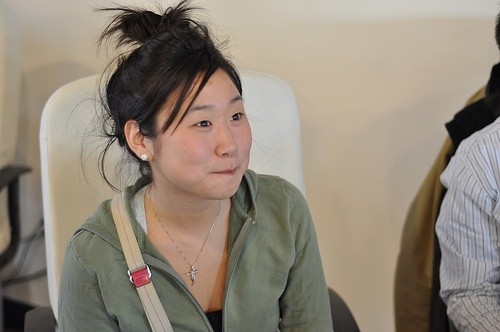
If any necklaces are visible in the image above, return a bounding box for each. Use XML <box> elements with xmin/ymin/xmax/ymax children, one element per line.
<box><xmin>147</xmin><ymin>183</ymin><xmax>224</xmax><ymax>287</ymax></box>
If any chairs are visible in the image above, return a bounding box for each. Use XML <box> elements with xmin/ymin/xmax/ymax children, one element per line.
<box><xmin>38</xmin><ymin>68</ymin><xmax>309</xmax><ymax>319</ymax></box>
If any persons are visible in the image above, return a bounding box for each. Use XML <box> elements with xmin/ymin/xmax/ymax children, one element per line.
<box><xmin>433</xmin><ymin>116</ymin><xmax>500</xmax><ymax>332</ymax></box>
<box><xmin>57</xmin><ymin>0</ymin><xmax>334</xmax><ymax>332</ymax></box>
<box><xmin>426</xmin><ymin>6</ymin><xmax>500</xmax><ymax>332</ymax></box>
